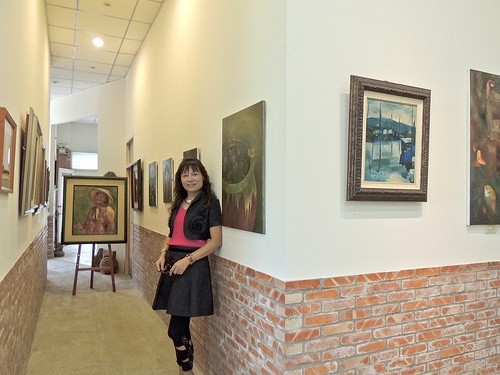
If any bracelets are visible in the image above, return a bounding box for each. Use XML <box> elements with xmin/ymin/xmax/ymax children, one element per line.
<box><xmin>186</xmin><ymin>254</ymin><xmax>194</xmax><ymax>264</ymax></box>
<box><xmin>160</xmin><ymin>249</ymin><xmax>168</xmax><ymax>254</ymax></box>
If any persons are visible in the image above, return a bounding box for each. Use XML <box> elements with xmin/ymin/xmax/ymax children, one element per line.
<box><xmin>152</xmin><ymin>159</ymin><xmax>222</xmax><ymax>375</ymax></box>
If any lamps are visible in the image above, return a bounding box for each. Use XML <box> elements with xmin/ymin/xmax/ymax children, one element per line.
<box><xmin>57</xmin><ymin>143</ymin><xmax>70</xmax><ymax>154</ymax></box>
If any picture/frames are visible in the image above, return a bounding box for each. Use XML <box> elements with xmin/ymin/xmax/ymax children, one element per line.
<box><xmin>61</xmin><ymin>176</ymin><xmax>127</xmax><ymax>245</ymax></box>
<box><xmin>346</xmin><ymin>74</ymin><xmax>431</xmax><ymax>202</ymax></box>
<box><xmin>0</xmin><ymin>106</ymin><xmax>50</xmax><ymax>216</ymax></box>
<box><xmin>131</xmin><ymin>159</ymin><xmax>142</xmax><ymax>211</ymax></box>
